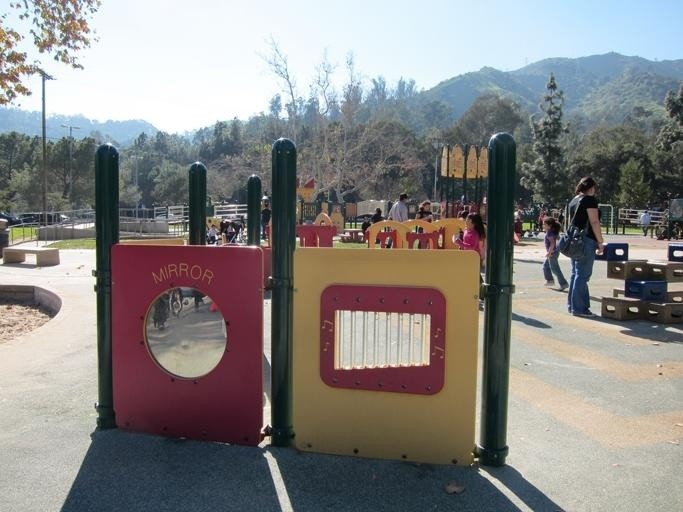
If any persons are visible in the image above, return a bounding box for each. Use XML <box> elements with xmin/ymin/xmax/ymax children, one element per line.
<box><xmin>386</xmin><ymin>200</ymin><xmax>395</xmax><ymax>248</ymax></box>
<box><xmin>388</xmin><ymin>194</ymin><xmax>408</xmax><ymax>222</ymax></box>
<box><xmin>568</xmin><ymin>177</ymin><xmax>604</xmax><ymax>317</ymax></box>
<box><xmin>208</xmin><ymin>216</ymin><xmax>245</xmax><ymax>244</ymax></box>
<box><xmin>362</xmin><ymin>217</ymin><xmax>371</xmax><ymax>242</ymax></box>
<box><xmin>414</xmin><ymin>202</ymin><xmax>432</xmax><ymax>249</ymax></box>
<box><xmin>538</xmin><ymin>208</ymin><xmax>565</xmax><ymax>233</ymax></box>
<box><xmin>371</xmin><ymin>208</ymin><xmax>382</xmax><ymax>243</ymax></box>
<box><xmin>543</xmin><ymin>218</ymin><xmax>569</xmax><ymax>291</ymax></box>
<box><xmin>261</xmin><ymin>204</ymin><xmax>271</xmax><ymax>239</ymax></box>
<box><xmin>514</xmin><ymin>205</ymin><xmax>524</xmax><ymax>241</ymax></box>
<box><xmin>640</xmin><ymin>211</ymin><xmax>651</xmax><ymax>236</ymax></box>
<box><xmin>454</xmin><ymin>200</ymin><xmax>488</xmax><ymax>309</ymax></box>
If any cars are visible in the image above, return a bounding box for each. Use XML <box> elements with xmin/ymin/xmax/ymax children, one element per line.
<box><xmin>0</xmin><ymin>212</ymin><xmax>70</xmax><ymax>225</ymax></box>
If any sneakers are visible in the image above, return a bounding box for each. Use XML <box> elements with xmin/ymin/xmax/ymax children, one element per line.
<box><xmin>557</xmin><ymin>282</ymin><xmax>570</xmax><ymax>291</ymax></box>
<box><xmin>544</xmin><ymin>280</ymin><xmax>556</xmax><ymax>287</ymax></box>
<box><xmin>568</xmin><ymin>309</ymin><xmax>596</xmax><ymax>317</ymax></box>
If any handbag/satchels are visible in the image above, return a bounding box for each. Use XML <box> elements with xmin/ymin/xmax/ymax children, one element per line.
<box><xmin>558</xmin><ymin>226</ymin><xmax>586</xmax><ymax>260</ymax></box>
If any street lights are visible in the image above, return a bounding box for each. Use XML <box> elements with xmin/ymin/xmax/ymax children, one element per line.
<box><xmin>60</xmin><ymin>124</ymin><xmax>80</xmax><ymax>192</ymax></box>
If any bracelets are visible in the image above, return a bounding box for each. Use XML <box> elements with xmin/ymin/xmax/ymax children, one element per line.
<box><xmin>598</xmin><ymin>238</ymin><xmax>604</xmax><ymax>244</ymax></box>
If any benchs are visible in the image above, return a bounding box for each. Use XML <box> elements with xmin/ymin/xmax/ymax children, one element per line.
<box><xmin>1</xmin><ymin>245</ymin><xmax>61</xmax><ymax>266</ymax></box>
